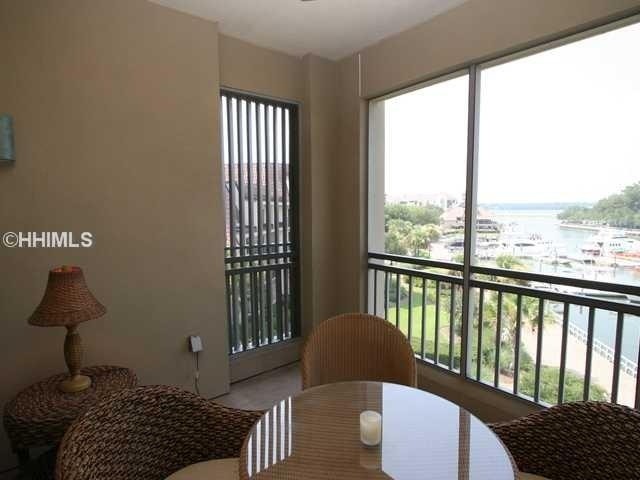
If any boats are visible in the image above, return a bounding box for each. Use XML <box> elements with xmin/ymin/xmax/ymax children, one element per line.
<box><xmin>476</xmin><ymin>236</ymin><xmax>640</xmax><ymax>304</ymax></box>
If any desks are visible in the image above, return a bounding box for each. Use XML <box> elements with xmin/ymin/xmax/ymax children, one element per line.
<box><xmin>2</xmin><ymin>361</ymin><xmax>142</xmax><ymax>476</ymax></box>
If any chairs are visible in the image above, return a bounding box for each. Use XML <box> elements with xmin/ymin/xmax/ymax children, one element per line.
<box><xmin>53</xmin><ymin>311</ymin><xmax>640</xmax><ymax>478</ymax></box>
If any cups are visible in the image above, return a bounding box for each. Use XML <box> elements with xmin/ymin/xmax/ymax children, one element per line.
<box><xmin>359</xmin><ymin>410</ymin><xmax>382</xmax><ymax>447</ymax></box>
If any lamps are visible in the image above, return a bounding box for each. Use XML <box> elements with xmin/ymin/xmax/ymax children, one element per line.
<box><xmin>25</xmin><ymin>263</ymin><xmax>107</xmax><ymax>394</ymax></box>
